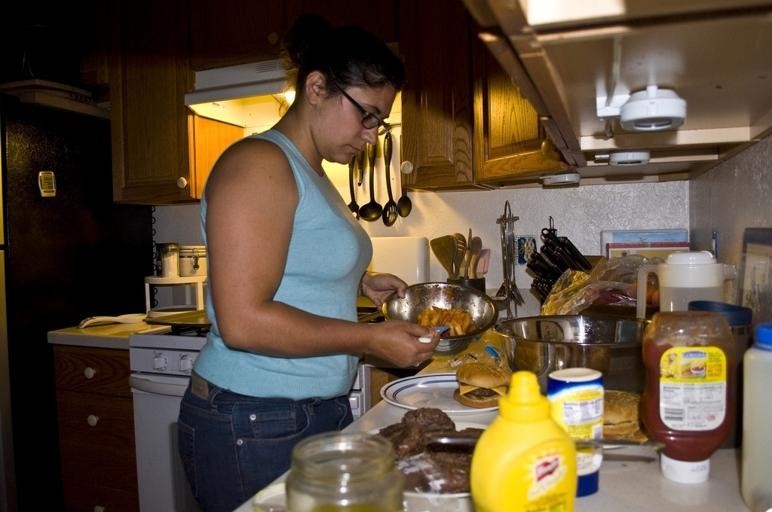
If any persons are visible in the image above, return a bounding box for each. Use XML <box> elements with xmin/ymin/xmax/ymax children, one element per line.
<box><xmin>178</xmin><ymin>16</ymin><xmax>440</xmax><ymax>512</ymax></box>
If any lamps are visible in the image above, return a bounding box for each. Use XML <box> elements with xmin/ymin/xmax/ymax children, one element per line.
<box><xmin>619</xmin><ymin>85</ymin><xmax>688</xmax><ymax>134</ymax></box>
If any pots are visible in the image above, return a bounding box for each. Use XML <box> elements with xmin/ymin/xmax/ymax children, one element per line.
<box><xmin>143</xmin><ymin>318</ymin><xmax>212</xmax><ymax>337</ymax></box>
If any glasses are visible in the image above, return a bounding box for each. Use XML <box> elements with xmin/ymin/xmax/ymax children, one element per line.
<box><xmin>332</xmin><ymin>83</ymin><xmax>391</xmax><ymax>136</ymax></box>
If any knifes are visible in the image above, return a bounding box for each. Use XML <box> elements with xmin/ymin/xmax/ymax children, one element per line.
<box><xmin>524</xmin><ymin>229</ymin><xmax>591</xmax><ymax>298</ymax></box>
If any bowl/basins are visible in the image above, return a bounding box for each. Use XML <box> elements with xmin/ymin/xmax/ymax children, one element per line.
<box><xmin>495</xmin><ymin>313</ymin><xmax>649</xmax><ymax>391</ymax></box>
<box><xmin>382</xmin><ymin>282</ymin><xmax>499</xmax><ymax>355</ymax></box>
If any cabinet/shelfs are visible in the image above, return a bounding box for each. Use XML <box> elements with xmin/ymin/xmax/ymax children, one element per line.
<box><xmin>399</xmin><ymin>5</ymin><xmax>562</xmax><ymax>192</ymax></box>
<box><xmin>48</xmin><ymin>331</ymin><xmax>133</xmax><ymax>511</ymax></box>
<box><xmin>187</xmin><ymin>3</ymin><xmax>398</xmax><ymax>94</ymax></box>
<box><xmin>110</xmin><ymin>13</ymin><xmax>237</xmax><ymax>206</ymax></box>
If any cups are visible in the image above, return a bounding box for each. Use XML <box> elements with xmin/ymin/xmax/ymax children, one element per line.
<box><xmin>636</xmin><ymin>252</ymin><xmax>738</xmax><ymax>317</ymax></box>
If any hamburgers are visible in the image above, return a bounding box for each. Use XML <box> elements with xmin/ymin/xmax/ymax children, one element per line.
<box><xmin>453</xmin><ymin>363</ymin><xmax>513</xmax><ymax>408</ymax></box>
<box><xmin>603</xmin><ymin>390</ymin><xmax>640</xmax><ymax>439</ymax></box>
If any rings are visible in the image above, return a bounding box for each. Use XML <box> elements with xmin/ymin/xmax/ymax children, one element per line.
<box><xmin>415</xmin><ymin>362</ymin><xmax>420</xmax><ymax>367</ymax></box>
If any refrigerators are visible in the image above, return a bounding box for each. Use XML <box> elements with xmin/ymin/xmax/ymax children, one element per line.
<box><xmin>129</xmin><ymin>374</ymin><xmax>362</xmax><ymax>512</ymax></box>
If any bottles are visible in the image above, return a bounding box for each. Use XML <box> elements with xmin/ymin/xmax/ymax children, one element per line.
<box><xmin>470</xmin><ymin>369</ymin><xmax>605</xmax><ymax>512</ymax></box>
<box><xmin>638</xmin><ymin>312</ymin><xmax>770</xmax><ymax>512</ymax></box>
<box><xmin>160</xmin><ymin>244</ymin><xmax>208</xmax><ymax>278</ymax></box>
<box><xmin>284</xmin><ymin>431</ymin><xmax>403</xmax><ymax>511</ymax></box>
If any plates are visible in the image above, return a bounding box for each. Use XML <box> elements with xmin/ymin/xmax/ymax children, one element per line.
<box><xmin>368</xmin><ymin>373</ymin><xmax>501</xmax><ymax>502</ymax></box>
